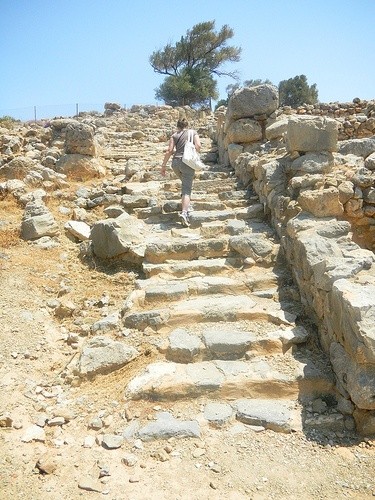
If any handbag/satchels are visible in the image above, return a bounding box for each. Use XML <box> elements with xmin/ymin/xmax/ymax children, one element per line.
<box><xmin>182</xmin><ymin>129</ymin><xmax>202</xmax><ymax>171</ymax></box>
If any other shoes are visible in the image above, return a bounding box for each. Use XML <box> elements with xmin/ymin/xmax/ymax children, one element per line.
<box><xmin>180</xmin><ymin>213</ymin><xmax>191</xmax><ymax>228</ymax></box>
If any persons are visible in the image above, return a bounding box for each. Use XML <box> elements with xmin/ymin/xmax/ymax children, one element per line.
<box><xmin>162</xmin><ymin>119</ymin><xmax>201</xmax><ymax>228</ymax></box>
